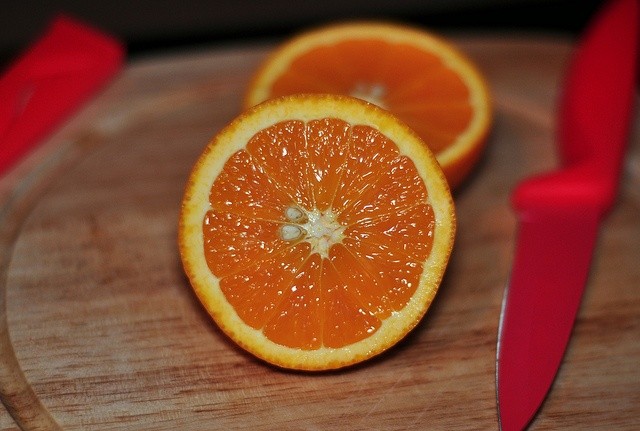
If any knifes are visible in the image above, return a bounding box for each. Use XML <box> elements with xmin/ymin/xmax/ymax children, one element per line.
<box><xmin>496</xmin><ymin>2</ymin><xmax>640</xmax><ymax>431</ymax></box>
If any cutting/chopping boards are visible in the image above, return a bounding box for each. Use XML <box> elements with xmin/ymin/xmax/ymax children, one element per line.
<box><xmin>0</xmin><ymin>33</ymin><xmax>640</xmax><ymax>429</ymax></box>
